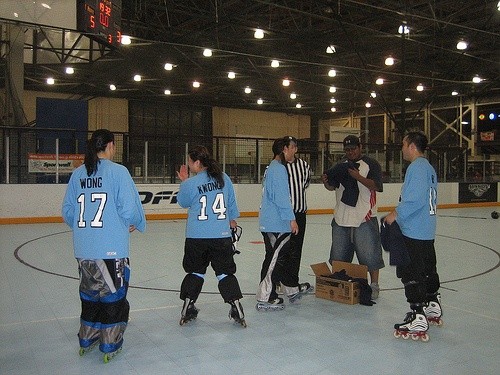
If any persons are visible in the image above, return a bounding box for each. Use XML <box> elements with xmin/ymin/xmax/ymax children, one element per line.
<box><xmin>257</xmin><ymin>136</ymin><xmax>312</xmax><ymax>304</ymax></box>
<box><xmin>384</xmin><ymin>132</ymin><xmax>443</xmax><ymax>343</ymax></box>
<box><xmin>61</xmin><ymin>129</ymin><xmax>146</xmax><ymax>363</ymax></box>
<box><xmin>321</xmin><ymin>135</ymin><xmax>386</xmax><ymax>300</ymax></box>
<box><xmin>177</xmin><ymin>145</ymin><xmax>246</xmax><ymax>326</ymax></box>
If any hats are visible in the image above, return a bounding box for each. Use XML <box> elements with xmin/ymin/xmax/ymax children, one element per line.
<box><xmin>343</xmin><ymin>135</ymin><xmax>359</xmax><ymax>150</ymax></box>
<box><xmin>283</xmin><ymin>136</ymin><xmax>297</xmax><ymax>144</ymax></box>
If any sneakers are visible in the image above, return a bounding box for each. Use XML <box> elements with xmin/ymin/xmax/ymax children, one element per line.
<box><xmin>370</xmin><ymin>283</ymin><xmax>379</xmax><ymax>300</ymax></box>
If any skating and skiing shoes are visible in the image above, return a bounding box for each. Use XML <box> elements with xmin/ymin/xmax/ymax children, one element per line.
<box><xmin>422</xmin><ymin>294</ymin><xmax>445</xmax><ymax>328</ymax></box>
<box><xmin>285</xmin><ymin>283</ymin><xmax>314</xmax><ymax>304</ymax></box>
<box><xmin>178</xmin><ymin>298</ymin><xmax>200</xmax><ymax>326</ymax></box>
<box><xmin>79</xmin><ymin>336</ymin><xmax>101</xmax><ymax>356</ymax></box>
<box><xmin>393</xmin><ymin>311</ymin><xmax>431</xmax><ymax>343</ymax></box>
<box><xmin>255</xmin><ymin>298</ymin><xmax>287</xmax><ymax>312</ymax></box>
<box><xmin>228</xmin><ymin>299</ymin><xmax>248</xmax><ymax>329</ymax></box>
<box><xmin>99</xmin><ymin>337</ymin><xmax>124</xmax><ymax>364</ymax></box>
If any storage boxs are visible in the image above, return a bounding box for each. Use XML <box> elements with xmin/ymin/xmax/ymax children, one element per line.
<box><xmin>309</xmin><ymin>259</ymin><xmax>369</xmax><ymax>305</ymax></box>
